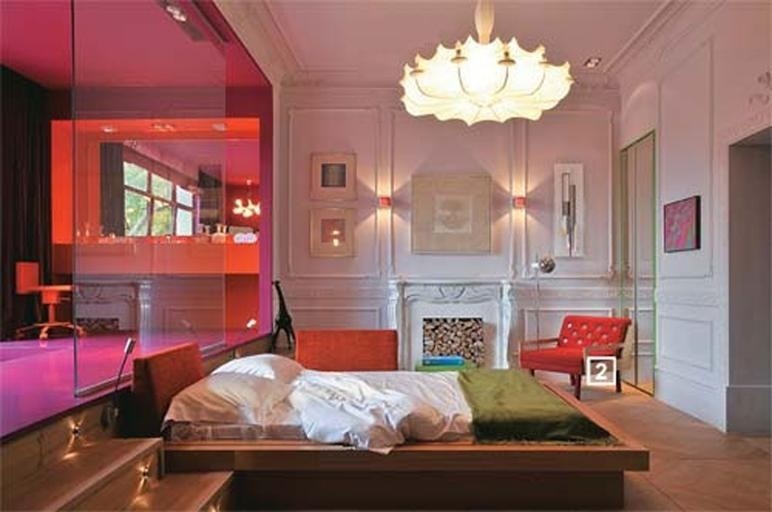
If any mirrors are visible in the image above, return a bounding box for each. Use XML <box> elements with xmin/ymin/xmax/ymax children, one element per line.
<box><xmin>77</xmin><ymin>130</ymin><xmax>261</xmax><ymax>239</ymax></box>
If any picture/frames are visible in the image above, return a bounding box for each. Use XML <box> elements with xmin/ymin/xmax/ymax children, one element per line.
<box><xmin>409</xmin><ymin>172</ymin><xmax>493</xmax><ymax>255</ymax></box>
<box><xmin>308</xmin><ymin>151</ymin><xmax>357</xmax><ymax>259</ymax></box>
<box><xmin>662</xmin><ymin>195</ymin><xmax>701</xmax><ymax>254</ymax></box>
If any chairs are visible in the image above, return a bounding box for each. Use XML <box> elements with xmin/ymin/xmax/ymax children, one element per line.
<box><xmin>519</xmin><ymin>314</ymin><xmax>632</xmax><ymax>402</ymax></box>
<box><xmin>11</xmin><ymin>261</ymin><xmax>85</xmax><ymax>340</ymax></box>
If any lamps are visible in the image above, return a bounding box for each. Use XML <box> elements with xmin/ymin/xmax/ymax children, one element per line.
<box><xmin>378</xmin><ymin>196</ymin><xmax>391</xmax><ymax>209</ymax></box>
<box><xmin>397</xmin><ymin>0</ymin><xmax>575</xmax><ymax>129</ymax></box>
<box><xmin>513</xmin><ymin>196</ymin><xmax>526</xmax><ymax>209</ymax></box>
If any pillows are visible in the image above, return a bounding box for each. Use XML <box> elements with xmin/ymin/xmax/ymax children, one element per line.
<box><xmin>162</xmin><ymin>350</ymin><xmax>304</xmax><ymax>422</ymax></box>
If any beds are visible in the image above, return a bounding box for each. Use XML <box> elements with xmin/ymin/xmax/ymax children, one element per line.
<box><xmin>131</xmin><ymin>344</ymin><xmax>649</xmax><ymax>511</ymax></box>
